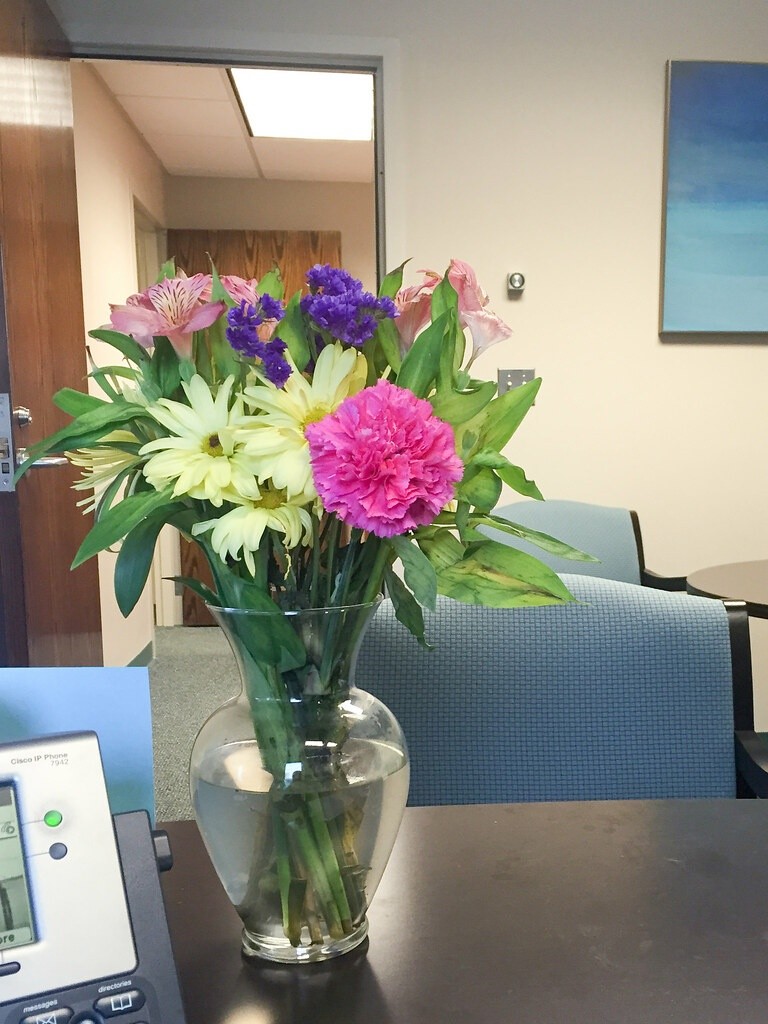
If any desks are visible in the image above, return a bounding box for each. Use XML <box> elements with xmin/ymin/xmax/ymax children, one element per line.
<box><xmin>152</xmin><ymin>799</ymin><xmax>768</xmax><ymax>1024</ymax></box>
<box><xmin>686</xmin><ymin>559</ymin><xmax>768</xmax><ymax>619</ymax></box>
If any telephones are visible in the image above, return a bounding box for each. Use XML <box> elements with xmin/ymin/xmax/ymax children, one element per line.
<box><xmin>0</xmin><ymin>732</ymin><xmax>187</xmax><ymax>1024</ymax></box>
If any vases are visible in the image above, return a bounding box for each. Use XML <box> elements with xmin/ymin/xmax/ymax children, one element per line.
<box><xmin>186</xmin><ymin>597</ymin><xmax>411</xmax><ymax>967</ymax></box>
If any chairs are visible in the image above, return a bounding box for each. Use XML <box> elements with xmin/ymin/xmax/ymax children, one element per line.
<box><xmin>350</xmin><ymin>575</ymin><xmax>768</xmax><ymax>806</ymax></box>
<box><xmin>475</xmin><ymin>498</ymin><xmax>686</xmax><ymax>593</ymax></box>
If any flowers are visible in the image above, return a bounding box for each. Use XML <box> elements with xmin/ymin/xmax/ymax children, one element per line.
<box><xmin>18</xmin><ymin>241</ymin><xmax>600</xmax><ymax>652</ymax></box>
